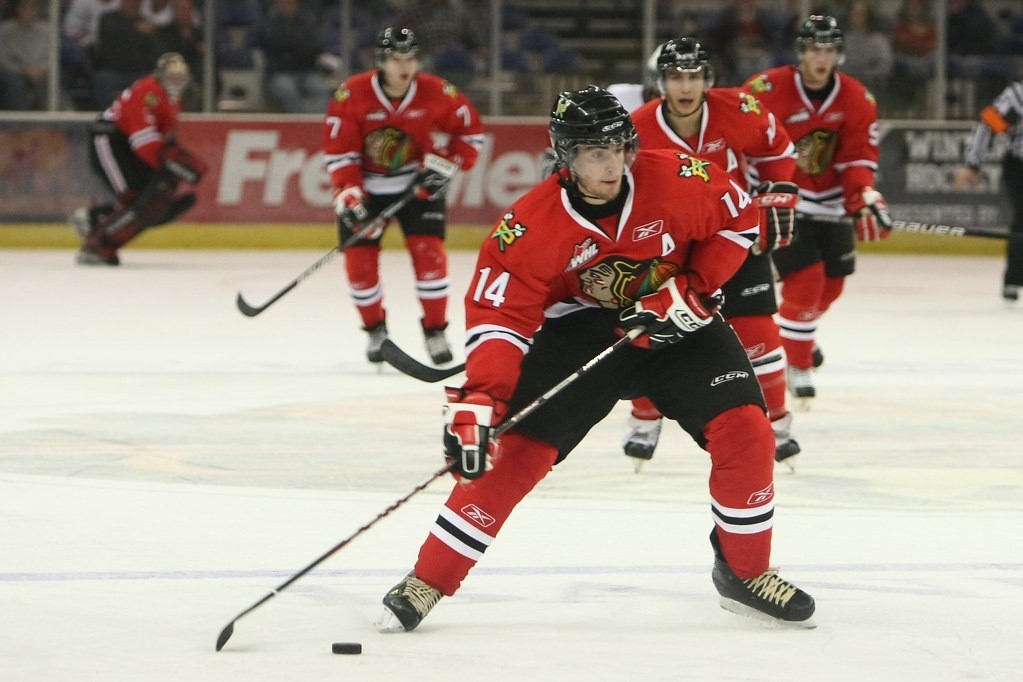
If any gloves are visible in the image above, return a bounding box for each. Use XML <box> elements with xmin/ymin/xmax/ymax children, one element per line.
<box><xmin>613</xmin><ymin>274</ymin><xmax>727</xmax><ymax>351</ymax></box>
<box><xmin>160</xmin><ymin>142</ymin><xmax>207</xmax><ymax>185</ymax></box>
<box><xmin>444</xmin><ymin>388</ymin><xmax>510</xmax><ymax>485</ymax></box>
<box><xmin>842</xmin><ymin>185</ymin><xmax>894</xmax><ymax>243</ymax></box>
<box><xmin>403</xmin><ymin>166</ymin><xmax>451</xmax><ymax>206</ymax></box>
<box><xmin>333</xmin><ymin>185</ymin><xmax>386</xmax><ymax>240</ymax></box>
<box><xmin>750</xmin><ymin>180</ymin><xmax>798</xmax><ymax>251</ymax></box>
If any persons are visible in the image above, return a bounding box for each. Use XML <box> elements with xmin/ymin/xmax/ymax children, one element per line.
<box><xmin>68</xmin><ymin>51</ymin><xmax>206</xmax><ymax>265</ymax></box>
<box><xmin>0</xmin><ymin>0</ymin><xmax>583</xmax><ymax>117</ymax></box>
<box><xmin>669</xmin><ymin>0</ymin><xmax>1023</xmax><ymax>116</ymax></box>
<box><xmin>322</xmin><ymin>27</ymin><xmax>484</xmax><ymax>367</ymax></box>
<box><xmin>622</xmin><ymin>37</ymin><xmax>803</xmax><ymax>478</ymax></box>
<box><xmin>740</xmin><ymin>16</ymin><xmax>894</xmax><ymax>413</ymax></box>
<box><xmin>372</xmin><ymin>84</ymin><xmax>816</xmax><ymax>636</ymax></box>
<box><xmin>957</xmin><ymin>82</ymin><xmax>1023</xmax><ymax>303</ymax></box>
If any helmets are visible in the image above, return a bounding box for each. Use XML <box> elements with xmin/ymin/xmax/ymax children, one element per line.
<box><xmin>156</xmin><ymin>51</ymin><xmax>191</xmax><ymax>98</ymax></box>
<box><xmin>793</xmin><ymin>14</ymin><xmax>846</xmax><ymax>66</ymax></box>
<box><xmin>548</xmin><ymin>83</ymin><xmax>639</xmax><ymax>162</ymax></box>
<box><xmin>373</xmin><ymin>24</ymin><xmax>420</xmax><ymax>61</ymax></box>
<box><xmin>646</xmin><ymin>36</ymin><xmax>715</xmax><ymax>99</ymax></box>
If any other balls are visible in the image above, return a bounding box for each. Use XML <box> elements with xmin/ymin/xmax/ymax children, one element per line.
<box><xmin>331</xmin><ymin>642</ymin><xmax>362</xmax><ymax>655</ymax></box>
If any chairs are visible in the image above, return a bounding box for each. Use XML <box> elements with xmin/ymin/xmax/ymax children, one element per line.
<box><xmin>0</xmin><ymin>0</ymin><xmax>1023</xmax><ymax>129</ymax></box>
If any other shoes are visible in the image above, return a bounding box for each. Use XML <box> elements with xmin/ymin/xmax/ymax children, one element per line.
<box><xmin>1001</xmin><ymin>284</ymin><xmax>1018</xmax><ymax>302</ymax></box>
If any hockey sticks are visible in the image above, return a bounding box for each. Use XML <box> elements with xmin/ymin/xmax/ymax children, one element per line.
<box><xmin>794</xmin><ymin>213</ymin><xmax>1023</xmax><ymax>242</ymax></box>
<box><xmin>235</xmin><ymin>186</ymin><xmax>420</xmax><ymax>319</ymax></box>
<box><xmin>214</xmin><ymin>324</ymin><xmax>651</xmax><ymax>653</ymax></box>
<box><xmin>380</xmin><ymin>338</ymin><xmax>466</xmax><ymax>384</ymax></box>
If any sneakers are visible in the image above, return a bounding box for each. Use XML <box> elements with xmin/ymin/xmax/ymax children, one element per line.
<box><xmin>770</xmin><ymin>411</ymin><xmax>801</xmax><ymax>474</ymax></box>
<box><xmin>374</xmin><ymin>568</ymin><xmax>444</xmax><ymax>632</ymax></box>
<box><xmin>74</xmin><ymin>199</ymin><xmax>115</xmax><ymax>238</ymax></box>
<box><xmin>787</xmin><ymin>366</ymin><xmax>815</xmax><ymax>411</ymax></box>
<box><xmin>810</xmin><ymin>344</ymin><xmax>823</xmax><ymax>370</ymax></box>
<box><xmin>709</xmin><ymin>525</ymin><xmax>817</xmax><ymax>630</ymax></box>
<box><xmin>75</xmin><ymin>233</ymin><xmax>121</xmax><ymax>266</ymax></box>
<box><xmin>363</xmin><ymin>308</ymin><xmax>388</xmax><ymax>371</ymax></box>
<box><xmin>421</xmin><ymin>317</ymin><xmax>453</xmax><ymax>369</ymax></box>
<box><xmin>622</xmin><ymin>411</ymin><xmax>663</xmax><ymax>474</ymax></box>
